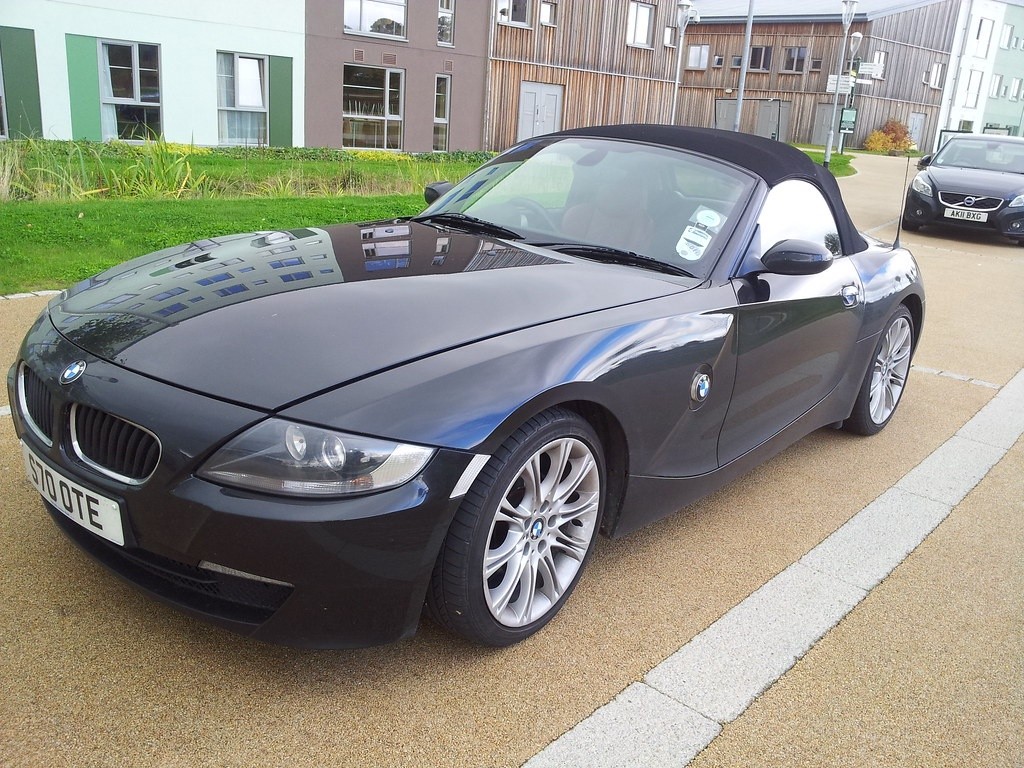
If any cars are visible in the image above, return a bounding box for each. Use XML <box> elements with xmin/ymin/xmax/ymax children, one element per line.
<box><xmin>902</xmin><ymin>133</ymin><xmax>1024</xmax><ymax>244</ymax></box>
<box><xmin>121</xmin><ymin>91</ymin><xmax>160</xmax><ymax>119</ymax></box>
<box><xmin>8</xmin><ymin>123</ymin><xmax>925</xmax><ymax>650</ymax></box>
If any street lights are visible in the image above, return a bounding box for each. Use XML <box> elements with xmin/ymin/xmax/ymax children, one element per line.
<box><xmin>823</xmin><ymin>0</ymin><xmax>861</xmax><ymax>169</ymax></box>
<box><xmin>670</xmin><ymin>0</ymin><xmax>694</xmax><ymax>124</ymax></box>
<box><xmin>837</xmin><ymin>31</ymin><xmax>863</xmax><ymax>154</ymax></box>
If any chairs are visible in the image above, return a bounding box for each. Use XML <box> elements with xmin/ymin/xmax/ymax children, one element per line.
<box><xmin>563</xmin><ymin>183</ymin><xmax>654</xmax><ymax>252</ymax></box>
